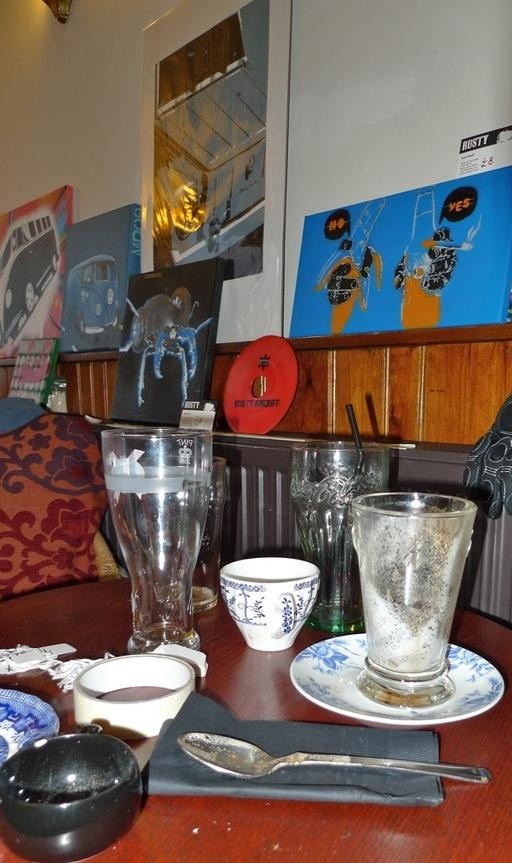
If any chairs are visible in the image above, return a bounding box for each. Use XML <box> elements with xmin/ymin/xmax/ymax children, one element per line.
<box><xmin>94</xmin><ymin>530</ymin><xmax>119</xmax><ymax>580</ymax></box>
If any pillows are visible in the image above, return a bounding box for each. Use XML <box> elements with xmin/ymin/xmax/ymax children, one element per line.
<box><xmin>0</xmin><ymin>396</ymin><xmax>108</xmax><ymax>601</ymax></box>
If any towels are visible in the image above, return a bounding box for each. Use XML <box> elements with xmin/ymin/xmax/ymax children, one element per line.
<box><xmin>146</xmin><ymin>693</ymin><xmax>443</xmax><ymax>806</ymax></box>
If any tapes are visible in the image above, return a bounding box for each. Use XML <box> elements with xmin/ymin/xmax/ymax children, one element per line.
<box><xmin>74</xmin><ymin>653</ymin><xmax>196</xmax><ymax>741</ymax></box>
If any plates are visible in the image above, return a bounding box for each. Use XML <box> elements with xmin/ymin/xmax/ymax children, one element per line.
<box><xmin>1</xmin><ymin>687</ymin><xmax>61</xmax><ymax>766</ymax></box>
<box><xmin>288</xmin><ymin>634</ymin><xmax>505</xmax><ymax>728</ymax></box>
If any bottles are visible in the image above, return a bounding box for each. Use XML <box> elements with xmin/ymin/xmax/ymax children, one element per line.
<box><xmin>400</xmin><ymin>185</ymin><xmax>440</xmax><ymax>327</ymax></box>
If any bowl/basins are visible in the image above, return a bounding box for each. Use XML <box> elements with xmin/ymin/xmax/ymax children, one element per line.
<box><xmin>1</xmin><ymin>722</ymin><xmax>144</xmax><ymax>862</ymax></box>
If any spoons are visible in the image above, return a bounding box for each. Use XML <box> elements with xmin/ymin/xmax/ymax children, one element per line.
<box><xmin>175</xmin><ymin>729</ymin><xmax>493</xmax><ymax>785</ymax></box>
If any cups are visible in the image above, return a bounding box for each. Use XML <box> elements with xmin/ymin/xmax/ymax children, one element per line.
<box><xmin>219</xmin><ymin>557</ymin><xmax>321</xmax><ymax>653</ymax></box>
<box><xmin>101</xmin><ymin>427</ymin><xmax>212</xmax><ymax>652</ymax></box>
<box><xmin>49</xmin><ymin>379</ymin><xmax>69</xmax><ymax>413</ymax></box>
<box><xmin>288</xmin><ymin>442</ymin><xmax>391</xmax><ymax>635</ymax></box>
<box><xmin>190</xmin><ymin>456</ymin><xmax>226</xmax><ymax>613</ymax></box>
<box><xmin>347</xmin><ymin>490</ymin><xmax>478</xmax><ymax>708</ymax></box>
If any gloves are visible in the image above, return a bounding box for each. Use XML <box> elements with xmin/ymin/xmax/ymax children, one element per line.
<box><xmin>461</xmin><ymin>395</ymin><xmax>512</xmax><ymax>520</ymax></box>
